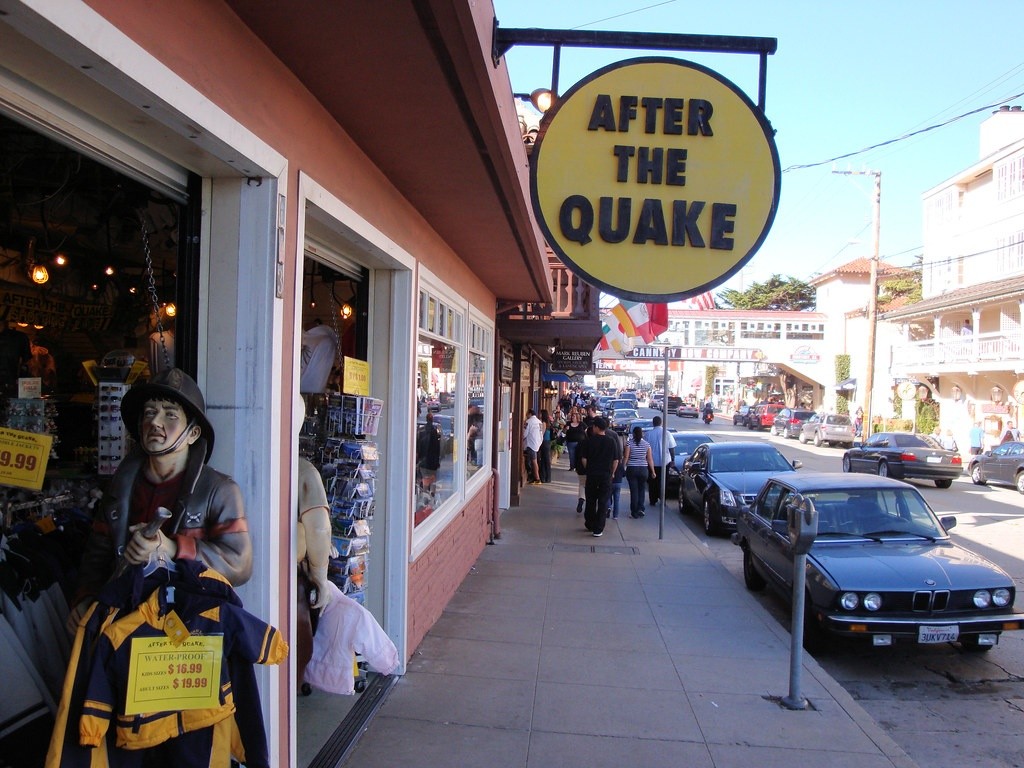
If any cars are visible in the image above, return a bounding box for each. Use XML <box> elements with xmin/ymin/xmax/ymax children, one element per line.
<box><xmin>426</xmin><ymin>391</ymin><xmax>455</xmax><ymax>412</ymax></box>
<box><xmin>967</xmin><ymin>442</ymin><xmax>1024</xmax><ymax>494</ymax></box>
<box><xmin>770</xmin><ymin>408</ymin><xmax>817</xmax><ymax>439</ymax></box>
<box><xmin>468</xmin><ymin>396</ymin><xmax>486</xmax><ymax>414</ymax></box>
<box><xmin>656</xmin><ymin>433</ymin><xmax>717</xmax><ymax>495</ymax></box>
<box><xmin>418</xmin><ymin>415</ymin><xmax>455</xmax><ymax>463</ymax></box>
<box><xmin>843</xmin><ymin>431</ymin><xmax>964</xmax><ymax>489</ymax></box>
<box><xmin>737</xmin><ymin>473</ymin><xmax>1024</xmax><ymax>654</ymax></box>
<box><xmin>582</xmin><ymin>385</ymin><xmax>700</xmax><ymax>419</ymax></box>
<box><xmin>609</xmin><ymin>408</ymin><xmax>645</xmax><ymax>435</ymax></box>
<box><xmin>679</xmin><ymin>441</ymin><xmax>803</xmax><ymax>534</ymax></box>
<box><xmin>733</xmin><ymin>406</ymin><xmax>759</xmax><ymax>427</ymax></box>
<box><xmin>749</xmin><ymin>404</ymin><xmax>787</xmax><ymax>430</ymax></box>
<box><xmin>799</xmin><ymin>412</ymin><xmax>856</xmax><ymax>447</ymax></box>
<box><xmin>623</xmin><ymin>419</ymin><xmax>654</xmax><ymax>440</ymax></box>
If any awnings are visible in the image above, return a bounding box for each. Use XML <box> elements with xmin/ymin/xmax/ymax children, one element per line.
<box><xmin>833</xmin><ymin>378</ymin><xmax>857</xmax><ymax>390</ymax></box>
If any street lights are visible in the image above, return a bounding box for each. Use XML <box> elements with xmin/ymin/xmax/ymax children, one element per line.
<box><xmin>833</xmin><ymin>168</ymin><xmax>881</xmax><ymax>439</ymax></box>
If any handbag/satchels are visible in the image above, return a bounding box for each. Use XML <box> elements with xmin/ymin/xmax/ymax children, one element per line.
<box><xmin>1</xmin><ymin>565</ymin><xmax>81</xmax><ymax>740</ymax></box>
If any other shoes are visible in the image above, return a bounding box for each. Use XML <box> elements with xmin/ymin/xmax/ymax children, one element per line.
<box><xmin>568</xmin><ymin>468</ymin><xmax>574</xmax><ymax>471</ymax></box>
<box><xmin>628</xmin><ymin>510</ymin><xmax>645</xmax><ymax>519</ymax></box>
<box><xmin>606</xmin><ymin>508</ymin><xmax>611</xmax><ymax>518</ymax></box>
<box><xmin>613</xmin><ymin>515</ymin><xmax>618</xmax><ymax>519</ymax></box>
<box><xmin>593</xmin><ymin>531</ymin><xmax>603</xmax><ymax>537</ymax></box>
<box><xmin>576</xmin><ymin>499</ymin><xmax>583</xmax><ymax>513</ymax></box>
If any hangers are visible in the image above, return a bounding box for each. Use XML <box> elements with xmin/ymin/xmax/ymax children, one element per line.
<box><xmin>7</xmin><ymin>491</ymin><xmax>86</xmax><ymax>526</ymax></box>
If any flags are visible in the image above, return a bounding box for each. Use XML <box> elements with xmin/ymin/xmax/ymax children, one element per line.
<box><xmin>597</xmin><ymin>302</ymin><xmax>669</xmax><ymax>353</ymax></box>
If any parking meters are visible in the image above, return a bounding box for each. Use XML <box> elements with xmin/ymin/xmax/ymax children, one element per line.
<box><xmin>779</xmin><ymin>493</ymin><xmax>819</xmax><ymax>711</ymax></box>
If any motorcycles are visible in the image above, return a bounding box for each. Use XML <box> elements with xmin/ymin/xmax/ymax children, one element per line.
<box><xmin>701</xmin><ymin>410</ymin><xmax>714</xmax><ymax>424</ymax></box>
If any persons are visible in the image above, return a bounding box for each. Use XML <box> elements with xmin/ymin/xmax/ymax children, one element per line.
<box><xmin>624</xmin><ymin>427</ymin><xmax>656</xmax><ymax>519</ymax></box>
<box><xmin>702</xmin><ymin>403</ymin><xmax>713</xmax><ymax>420</ymax></box>
<box><xmin>928</xmin><ymin>426</ymin><xmax>958</xmax><ymax>452</ymax></box>
<box><xmin>101</xmin><ymin>369</ymin><xmax>253</xmax><ymax>587</ymax></box>
<box><xmin>720</xmin><ymin>334</ymin><xmax>729</xmax><ymax>347</ymax></box>
<box><xmin>552</xmin><ymin>382</ymin><xmax>626</xmax><ymax>536</ymax></box>
<box><xmin>1000</xmin><ymin>421</ymin><xmax>1021</xmax><ymax>443</ymax></box>
<box><xmin>741</xmin><ymin>399</ymin><xmax>813</xmax><ymax>410</ymax></box>
<box><xmin>298</xmin><ymin>394</ymin><xmax>331</xmax><ymax>608</ymax></box>
<box><xmin>961</xmin><ymin>319</ymin><xmax>973</xmax><ymax>335</ymax></box>
<box><xmin>467</xmin><ymin>405</ymin><xmax>484</xmax><ymax>467</ymax></box>
<box><xmin>648</xmin><ymin>416</ymin><xmax>677</xmax><ymax>506</ymax></box>
<box><xmin>854</xmin><ymin>407</ymin><xmax>864</xmax><ymax>437</ymax></box>
<box><xmin>523</xmin><ymin>408</ymin><xmax>552</xmax><ymax>484</ymax></box>
<box><xmin>966</xmin><ymin>417</ymin><xmax>985</xmax><ymax>455</ymax></box>
<box><xmin>417</xmin><ymin>414</ymin><xmax>442</xmax><ymax>505</ymax></box>
